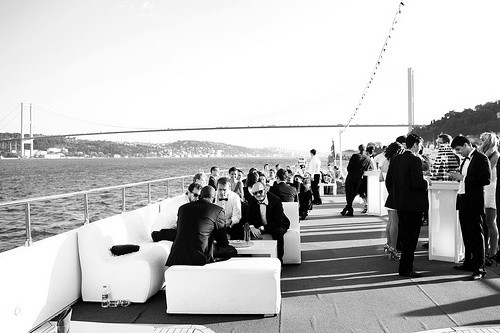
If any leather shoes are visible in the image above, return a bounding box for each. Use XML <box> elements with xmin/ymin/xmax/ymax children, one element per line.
<box><xmin>409</xmin><ymin>273</ymin><xmax>422</xmax><ymax>277</ymax></box>
<box><xmin>471</xmin><ymin>273</ymin><xmax>483</xmax><ymax>280</ymax></box>
<box><xmin>453</xmin><ymin>264</ymin><xmax>471</xmax><ymax>270</ymax></box>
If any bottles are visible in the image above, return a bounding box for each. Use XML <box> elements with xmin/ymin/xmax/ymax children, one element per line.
<box><xmin>244</xmin><ymin>223</ymin><xmax>251</xmax><ymax>248</ymax></box>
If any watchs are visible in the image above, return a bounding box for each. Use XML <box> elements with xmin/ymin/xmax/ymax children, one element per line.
<box><xmin>260</xmin><ymin>226</ymin><xmax>264</xmax><ymax>232</ymax></box>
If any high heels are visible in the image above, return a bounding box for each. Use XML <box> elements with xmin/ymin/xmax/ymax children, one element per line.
<box><xmin>389</xmin><ymin>247</ymin><xmax>401</xmax><ymax>261</ymax></box>
<box><xmin>485</xmin><ymin>251</ymin><xmax>498</xmax><ymax>266</ymax></box>
<box><xmin>384</xmin><ymin>244</ymin><xmax>390</xmax><ymax>257</ymax></box>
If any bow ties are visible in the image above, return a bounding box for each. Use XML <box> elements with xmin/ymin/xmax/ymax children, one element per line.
<box><xmin>219</xmin><ymin>198</ymin><xmax>228</xmax><ymax>201</ymax></box>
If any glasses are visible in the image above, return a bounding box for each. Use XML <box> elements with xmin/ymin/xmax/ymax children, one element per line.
<box><xmin>253</xmin><ymin>190</ymin><xmax>264</xmax><ymax>195</ymax></box>
<box><xmin>191</xmin><ymin>192</ymin><xmax>200</xmax><ymax>197</ymax></box>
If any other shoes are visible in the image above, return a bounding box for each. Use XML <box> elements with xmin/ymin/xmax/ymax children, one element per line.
<box><xmin>313</xmin><ymin>200</ymin><xmax>322</xmax><ymax>205</ymax></box>
<box><xmin>361</xmin><ymin>205</ymin><xmax>367</xmax><ymax>213</ymax></box>
<box><xmin>341</xmin><ymin>211</ymin><xmax>353</xmax><ymax>216</ymax></box>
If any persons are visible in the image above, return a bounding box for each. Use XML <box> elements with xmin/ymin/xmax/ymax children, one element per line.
<box><xmin>308</xmin><ymin>149</ymin><xmax>323</xmax><ymax>205</ymax></box>
<box><xmin>450</xmin><ymin>135</ymin><xmax>492</xmax><ymax>281</ymax></box>
<box><xmin>384</xmin><ymin>134</ymin><xmax>433</xmax><ymax>279</ymax></box>
<box><xmin>362</xmin><ymin>131</ymin><xmax>500</xmax><ymax>267</ymax></box>
<box><xmin>340</xmin><ymin>143</ymin><xmax>374</xmax><ymax>216</ymax></box>
<box><xmin>175</xmin><ymin>157</ymin><xmax>346</xmax><ymax>253</ymax></box>
<box><xmin>165</xmin><ymin>185</ymin><xmax>239</xmax><ymax>266</ymax></box>
<box><xmin>238</xmin><ymin>182</ymin><xmax>291</xmax><ymax>273</ymax></box>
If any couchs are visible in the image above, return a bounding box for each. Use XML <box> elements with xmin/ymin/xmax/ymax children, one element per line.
<box><xmin>78</xmin><ymin>195</ymin><xmax>301</xmax><ymax>305</ymax></box>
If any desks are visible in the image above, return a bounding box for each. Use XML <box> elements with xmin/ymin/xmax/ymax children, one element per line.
<box><xmin>365</xmin><ymin>171</ymin><xmax>391</xmax><ymax>216</ymax></box>
<box><xmin>428</xmin><ymin>180</ymin><xmax>461</xmax><ymax>263</ymax></box>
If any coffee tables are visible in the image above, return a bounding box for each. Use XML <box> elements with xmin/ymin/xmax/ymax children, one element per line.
<box><xmin>306</xmin><ymin>183</ymin><xmax>337</xmax><ymax>197</ymax></box>
<box><xmin>226</xmin><ymin>239</ymin><xmax>277</xmax><ymax>260</ymax></box>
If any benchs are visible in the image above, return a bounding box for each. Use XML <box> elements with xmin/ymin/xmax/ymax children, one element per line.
<box><xmin>165</xmin><ymin>256</ymin><xmax>281</xmax><ymax>316</ymax></box>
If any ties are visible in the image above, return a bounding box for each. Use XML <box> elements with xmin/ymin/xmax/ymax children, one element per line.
<box><xmin>457</xmin><ymin>157</ymin><xmax>469</xmax><ymax>182</ymax></box>
